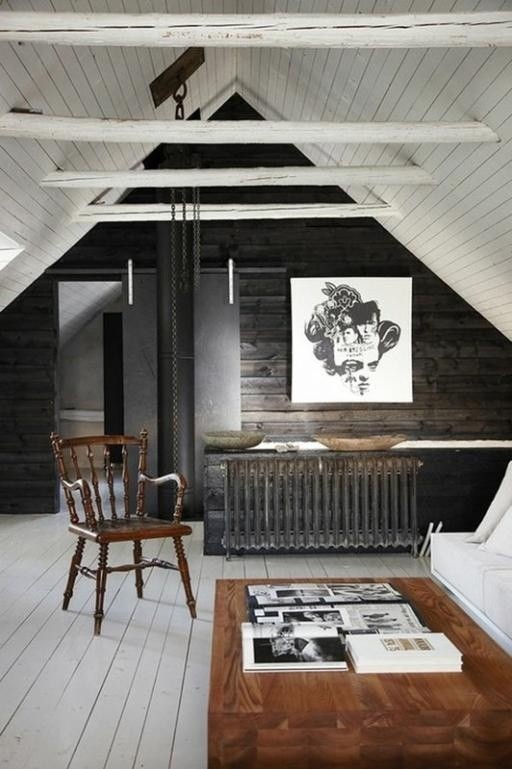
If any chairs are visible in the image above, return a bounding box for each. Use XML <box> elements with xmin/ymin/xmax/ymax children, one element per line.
<box><xmin>49</xmin><ymin>426</ymin><xmax>198</xmax><ymax>637</ymax></box>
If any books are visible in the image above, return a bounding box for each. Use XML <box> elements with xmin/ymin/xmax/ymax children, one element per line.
<box><xmin>239</xmin><ymin>581</ymin><xmax>464</xmax><ymax>675</ymax></box>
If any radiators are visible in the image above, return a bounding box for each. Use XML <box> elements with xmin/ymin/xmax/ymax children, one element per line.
<box><xmin>220</xmin><ymin>455</ymin><xmax>424</xmax><ymax>561</ymax></box>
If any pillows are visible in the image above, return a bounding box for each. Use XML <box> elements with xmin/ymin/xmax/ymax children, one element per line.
<box><xmin>464</xmin><ymin>459</ymin><xmax>512</xmax><ymax>557</ymax></box>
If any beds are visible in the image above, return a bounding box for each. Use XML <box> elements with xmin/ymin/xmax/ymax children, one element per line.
<box><xmin>430</xmin><ymin>532</ymin><xmax>512</xmax><ymax>658</ymax></box>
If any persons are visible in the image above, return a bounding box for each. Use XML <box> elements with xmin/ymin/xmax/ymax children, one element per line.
<box><xmin>342</xmin><ymin>326</ymin><xmax>358</xmax><ymax>343</ymax></box>
<box><xmin>353</xmin><ymin>301</ymin><xmax>379</xmax><ymax>342</ymax></box>
<box><xmin>336</xmin><ymin>346</ymin><xmax>380</xmax><ymax>397</ymax></box>
